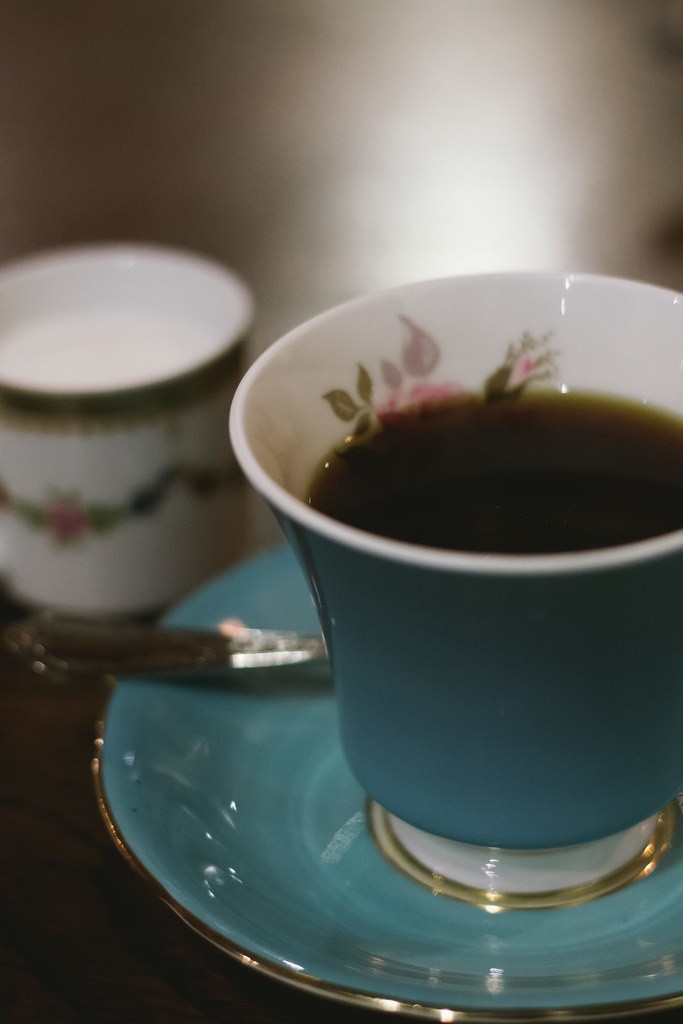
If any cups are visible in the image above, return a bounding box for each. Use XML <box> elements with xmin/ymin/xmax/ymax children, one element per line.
<box><xmin>0</xmin><ymin>245</ymin><xmax>255</xmax><ymax>614</ymax></box>
<box><xmin>228</xmin><ymin>273</ymin><xmax>683</xmax><ymax>905</ymax></box>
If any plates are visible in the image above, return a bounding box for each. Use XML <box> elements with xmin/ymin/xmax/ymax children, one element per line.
<box><xmin>95</xmin><ymin>547</ymin><xmax>683</xmax><ymax>1022</ymax></box>
